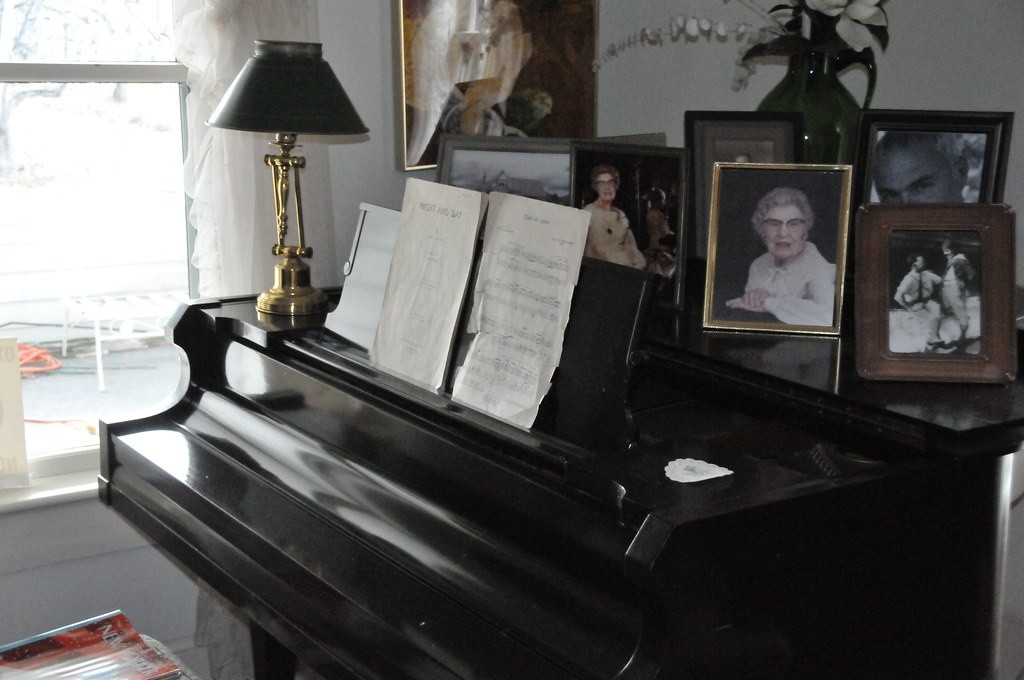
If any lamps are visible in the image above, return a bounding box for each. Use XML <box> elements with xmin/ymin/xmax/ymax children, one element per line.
<box><xmin>205</xmin><ymin>38</ymin><xmax>369</xmax><ymax>315</ymax></box>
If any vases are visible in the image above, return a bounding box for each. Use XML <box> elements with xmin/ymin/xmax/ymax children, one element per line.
<box><xmin>751</xmin><ymin>37</ymin><xmax>864</xmax><ymax>208</ymax></box>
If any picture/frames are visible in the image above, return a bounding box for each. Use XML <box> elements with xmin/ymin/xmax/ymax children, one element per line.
<box><xmin>684</xmin><ymin>108</ymin><xmax>1015</xmax><ymax>385</ymax></box>
<box><xmin>397</xmin><ymin>0</ymin><xmax>599</xmax><ymax>172</ymax></box>
<box><xmin>435</xmin><ymin>135</ymin><xmax>684</xmax><ymax>292</ymax></box>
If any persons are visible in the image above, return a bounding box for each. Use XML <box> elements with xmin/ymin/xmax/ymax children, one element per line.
<box><xmin>874</xmin><ymin>131</ymin><xmax>969</xmax><ymax>205</ymax></box>
<box><xmin>725</xmin><ymin>187</ymin><xmax>837</xmax><ymax>327</ymax></box>
<box><xmin>584</xmin><ymin>167</ymin><xmax>677</xmax><ymax>277</ymax></box>
<box><xmin>895</xmin><ymin>239</ymin><xmax>981</xmax><ymax>355</ymax></box>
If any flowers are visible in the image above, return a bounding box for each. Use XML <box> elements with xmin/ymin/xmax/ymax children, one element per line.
<box><xmin>603</xmin><ymin>0</ymin><xmax>890</xmax><ymax>54</ymax></box>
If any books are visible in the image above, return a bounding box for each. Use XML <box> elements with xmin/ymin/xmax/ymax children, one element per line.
<box><xmin>0</xmin><ymin>609</ymin><xmax>181</xmax><ymax>680</ymax></box>
<box><xmin>370</xmin><ymin>176</ymin><xmax>490</xmax><ymax>392</ymax></box>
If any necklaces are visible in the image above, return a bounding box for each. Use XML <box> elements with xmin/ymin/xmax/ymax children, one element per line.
<box><xmin>596</xmin><ymin>205</ymin><xmax>612</xmax><ymax>234</ymax></box>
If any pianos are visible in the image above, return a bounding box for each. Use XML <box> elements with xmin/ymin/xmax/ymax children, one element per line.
<box><xmin>97</xmin><ymin>284</ymin><xmax>1024</xmax><ymax>680</ymax></box>
